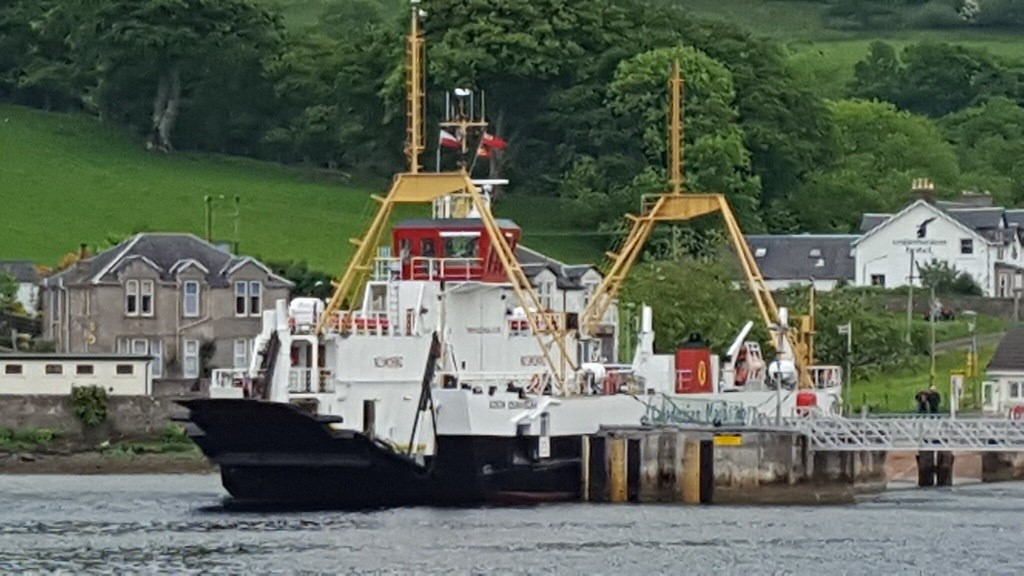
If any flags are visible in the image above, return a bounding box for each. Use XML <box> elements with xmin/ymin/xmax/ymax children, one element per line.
<box><xmin>483</xmin><ymin>134</ymin><xmax>506</xmax><ymax>149</ymax></box>
<box><xmin>480</xmin><ymin>148</ymin><xmax>493</xmax><ymax>158</ymax></box>
<box><xmin>441</xmin><ymin>131</ymin><xmax>460</xmax><ymax>148</ymax></box>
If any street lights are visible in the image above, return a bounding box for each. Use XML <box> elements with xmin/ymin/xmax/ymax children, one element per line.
<box><xmin>862</xmin><ymin>255</ymin><xmax>889</xmax><ymax>314</ymax></box>
<box><xmin>963</xmin><ymin>309</ymin><xmax>980</xmax><ymax>407</ymax></box>
<box><xmin>929</xmin><ymin>274</ymin><xmax>946</xmax><ymax>385</ymax></box>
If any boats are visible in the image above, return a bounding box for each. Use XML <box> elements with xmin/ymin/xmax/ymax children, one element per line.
<box><xmin>169</xmin><ymin>0</ymin><xmax>621</xmax><ymax>510</ymax></box>
<box><xmin>480</xmin><ymin>398</ymin><xmax>599</xmax><ymax>504</ymax></box>
<box><xmin>433</xmin><ymin>54</ymin><xmax>844</xmax><ymax>513</ymax></box>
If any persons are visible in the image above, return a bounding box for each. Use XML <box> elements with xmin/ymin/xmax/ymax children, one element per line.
<box><xmin>915</xmin><ymin>385</ymin><xmax>941</xmax><ymax>413</ymax></box>
